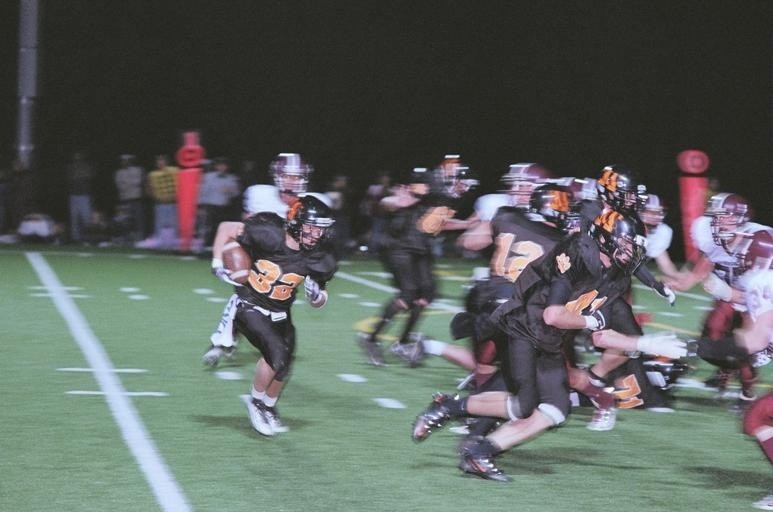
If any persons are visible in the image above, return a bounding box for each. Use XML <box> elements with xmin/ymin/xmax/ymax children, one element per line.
<box><xmin>113</xmin><ymin>153</ymin><xmax>145</xmax><ymax>240</ymax></box>
<box><xmin>147</xmin><ymin>151</ymin><xmax>180</xmax><ymax>231</ymax></box>
<box><xmin>198</xmin><ymin>151</ymin><xmax>773</xmax><ymax>484</ymax></box>
<box><xmin>67</xmin><ymin>151</ymin><xmax>93</xmax><ymax>241</ymax></box>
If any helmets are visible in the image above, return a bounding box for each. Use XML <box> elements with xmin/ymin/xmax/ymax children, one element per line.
<box><xmin>640</xmin><ymin>353</ymin><xmax>687</xmax><ymax>388</ymax></box>
<box><xmin>436</xmin><ymin>152</ymin><xmax>480</xmax><ymax>199</ymax></box>
<box><xmin>269</xmin><ymin>153</ymin><xmax>313</xmax><ymax>193</ymax></box>
<box><xmin>505</xmin><ymin>162</ymin><xmax>666</xmax><ymax>274</ymax></box>
<box><xmin>288</xmin><ymin>193</ymin><xmax>335</xmax><ymax>248</ymax></box>
<box><xmin>703</xmin><ymin>194</ymin><xmax>753</xmax><ymax>244</ymax></box>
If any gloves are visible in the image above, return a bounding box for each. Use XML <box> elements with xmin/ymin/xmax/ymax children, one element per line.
<box><xmin>702</xmin><ymin>272</ymin><xmax>731</xmax><ymax>303</ymax></box>
<box><xmin>210</xmin><ymin>256</ymin><xmax>244</xmax><ymax>286</ymax></box>
<box><xmin>585</xmin><ymin>306</ymin><xmax>615</xmax><ymax>331</ymax></box>
<box><xmin>652</xmin><ymin>280</ymin><xmax>679</xmax><ymax>306</ymax></box>
<box><xmin>302</xmin><ymin>274</ymin><xmax>323</xmax><ymax>306</ymax></box>
<box><xmin>636</xmin><ymin>332</ymin><xmax>688</xmax><ymax>360</ymax></box>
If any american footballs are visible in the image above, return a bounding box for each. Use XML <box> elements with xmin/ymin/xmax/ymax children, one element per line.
<box><xmin>222</xmin><ymin>238</ymin><xmax>251</xmax><ymax>284</ymax></box>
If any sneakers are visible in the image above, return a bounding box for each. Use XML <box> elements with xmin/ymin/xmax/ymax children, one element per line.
<box><xmin>201</xmin><ymin>345</ymin><xmax>226</xmax><ymax>367</ymax></box>
<box><xmin>585</xmin><ymin>394</ymin><xmax>616</xmax><ymax>431</ymax></box>
<box><xmin>750</xmin><ymin>493</ymin><xmax>772</xmax><ymax>511</ymax></box>
<box><xmin>411</xmin><ymin>372</ymin><xmax>511</xmax><ymax>483</ymax></box>
<box><xmin>245</xmin><ymin>396</ymin><xmax>290</xmax><ymax>435</ymax></box>
<box><xmin>704</xmin><ymin>366</ymin><xmax>760</xmax><ymax>400</ymax></box>
<box><xmin>355</xmin><ymin>328</ymin><xmax>433</xmax><ymax>369</ymax></box>
